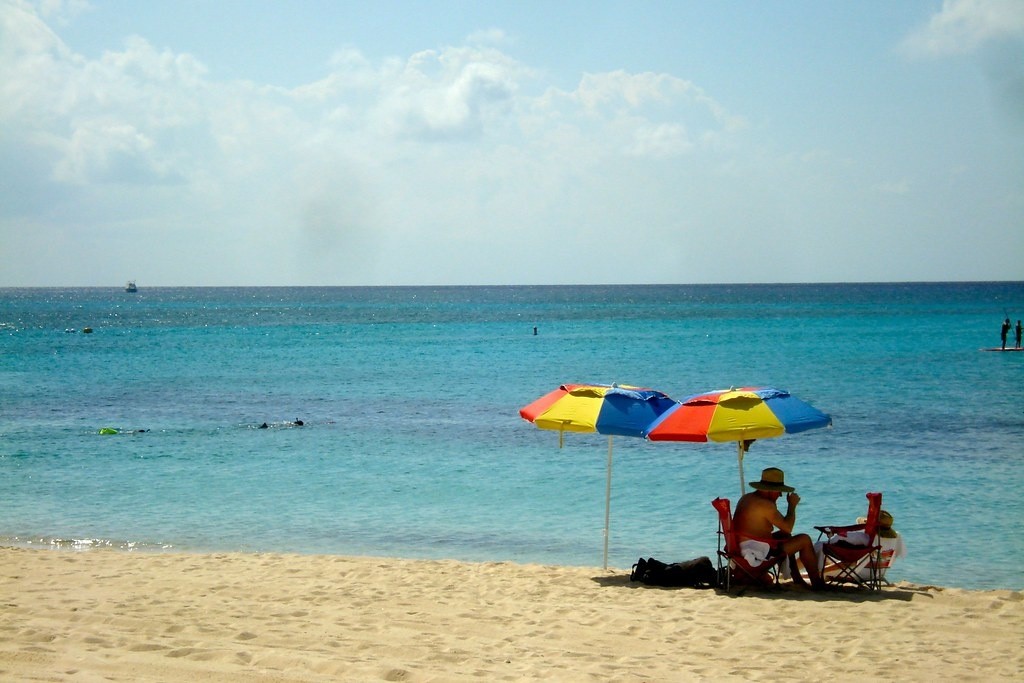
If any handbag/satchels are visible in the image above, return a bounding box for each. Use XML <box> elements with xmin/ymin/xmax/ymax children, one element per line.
<box><xmin>630</xmin><ymin>556</ymin><xmax>716</xmax><ymax>589</ymax></box>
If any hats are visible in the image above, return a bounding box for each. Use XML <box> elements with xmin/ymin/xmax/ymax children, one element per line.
<box><xmin>748</xmin><ymin>466</ymin><xmax>796</xmax><ymax>493</ymax></box>
<box><xmin>857</xmin><ymin>509</ymin><xmax>897</xmax><ymax>538</ymax></box>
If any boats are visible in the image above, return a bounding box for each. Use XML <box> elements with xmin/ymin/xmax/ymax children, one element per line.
<box><xmin>126</xmin><ymin>281</ymin><xmax>137</xmax><ymax>293</ymax></box>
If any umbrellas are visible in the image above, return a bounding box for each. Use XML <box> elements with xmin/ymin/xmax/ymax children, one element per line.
<box><xmin>642</xmin><ymin>383</ymin><xmax>833</xmax><ymax>494</ymax></box>
<box><xmin>517</xmin><ymin>381</ymin><xmax>676</xmax><ymax>568</ymax></box>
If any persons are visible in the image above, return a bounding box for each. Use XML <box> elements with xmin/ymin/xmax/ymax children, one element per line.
<box><xmin>1015</xmin><ymin>320</ymin><xmax>1024</xmax><ymax>349</ymax></box>
<box><xmin>1000</xmin><ymin>319</ymin><xmax>1011</xmax><ymax>348</ymax></box>
<box><xmin>796</xmin><ymin>511</ymin><xmax>895</xmax><ymax>568</ymax></box>
<box><xmin>732</xmin><ymin>467</ymin><xmax>829</xmax><ymax>592</ymax></box>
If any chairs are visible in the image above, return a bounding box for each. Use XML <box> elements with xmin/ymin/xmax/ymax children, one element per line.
<box><xmin>711</xmin><ymin>496</ymin><xmax>787</xmax><ymax>594</ymax></box>
<box><xmin>801</xmin><ymin>491</ymin><xmax>907</xmax><ymax>595</ymax></box>
